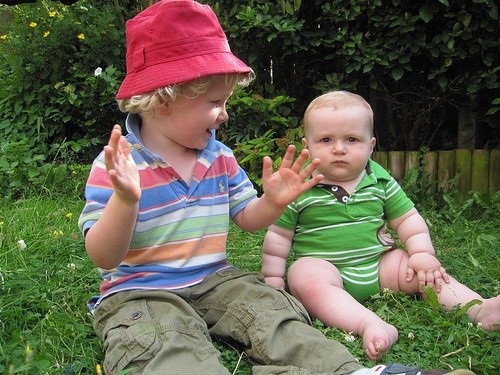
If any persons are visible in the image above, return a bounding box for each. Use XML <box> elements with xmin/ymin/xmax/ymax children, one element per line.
<box><xmin>77</xmin><ymin>0</ymin><xmax>476</xmax><ymax>375</ymax></box>
<box><xmin>258</xmin><ymin>88</ymin><xmax>500</xmax><ymax>361</ymax></box>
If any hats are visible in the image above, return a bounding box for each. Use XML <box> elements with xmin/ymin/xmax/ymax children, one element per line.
<box><xmin>115</xmin><ymin>0</ymin><xmax>251</xmax><ymax>99</ymax></box>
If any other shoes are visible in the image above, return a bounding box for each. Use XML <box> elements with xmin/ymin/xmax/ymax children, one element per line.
<box><xmin>366</xmin><ymin>362</ymin><xmax>477</xmax><ymax>375</ymax></box>
